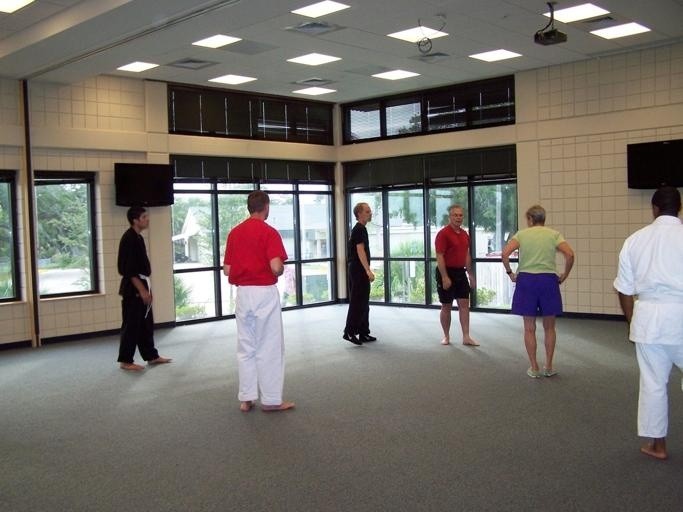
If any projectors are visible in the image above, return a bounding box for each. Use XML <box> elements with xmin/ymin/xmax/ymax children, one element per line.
<box><xmin>534</xmin><ymin>28</ymin><xmax>567</xmax><ymax>46</ymax></box>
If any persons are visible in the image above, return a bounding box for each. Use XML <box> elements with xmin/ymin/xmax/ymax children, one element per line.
<box><xmin>342</xmin><ymin>202</ymin><xmax>377</xmax><ymax>345</ymax></box>
<box><xmin>501</xmin><ymin>204</ymin><xmax>575</xmax><ymax>379</ymax></box>
<box><xmin>222</xmin><ymin>190</ymin><xmax>296</xmax><ymax>412</ymax></box>
<box><xmin>435</xmin><ymin>204</ymin><xmax>481</xmax><ymax>347</ymax></box>
<box><xmin>614</xmin><ymin>185</ymin><xmax>683</xmax><ymax>459</ymax></box>
<box><xmin>112</xmin><ymin>205</ymin><xmax>173</xmax><ymax>371</ymax></box>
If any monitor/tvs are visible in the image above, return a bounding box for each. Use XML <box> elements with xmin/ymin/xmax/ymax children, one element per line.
<box><xmin>115</xmin><ymin>163</ymin><xmax>174</xmax><ymax>207</ymax></box>
<box><xmin>627</xmin><ymin>139</ymin><xmax>683</xmax><ymax>189</ymax></box>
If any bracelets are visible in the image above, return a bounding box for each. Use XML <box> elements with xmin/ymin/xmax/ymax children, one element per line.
<box><xmin>366</xmin><ymin>271</ymin><xmax>370</xmax><ymax>274</ymax></box>
<box><xmin>506</xmin><ymin>269</ymin><xmax>512</xmax><ymax>275</ymax></box>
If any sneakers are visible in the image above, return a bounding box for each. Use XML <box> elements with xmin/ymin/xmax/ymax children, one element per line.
<box><xmin>359</xmin><ymin>334</ymin><xmax>375</xmax><ymax>341</ymax></box>
<box><xmin>526</xmin><ymin>367</ymin><xmax>540</xmax><ymax>378</ymax></box>
<box><xmin>343</xmin><ymin>332</ymin><xmax>362</xmax><ymax>345</ymax></box>
<box><xmin>543</xmin><ymin>368</ymin><xmax>560</xmax><ymax>376</ymax></box>
